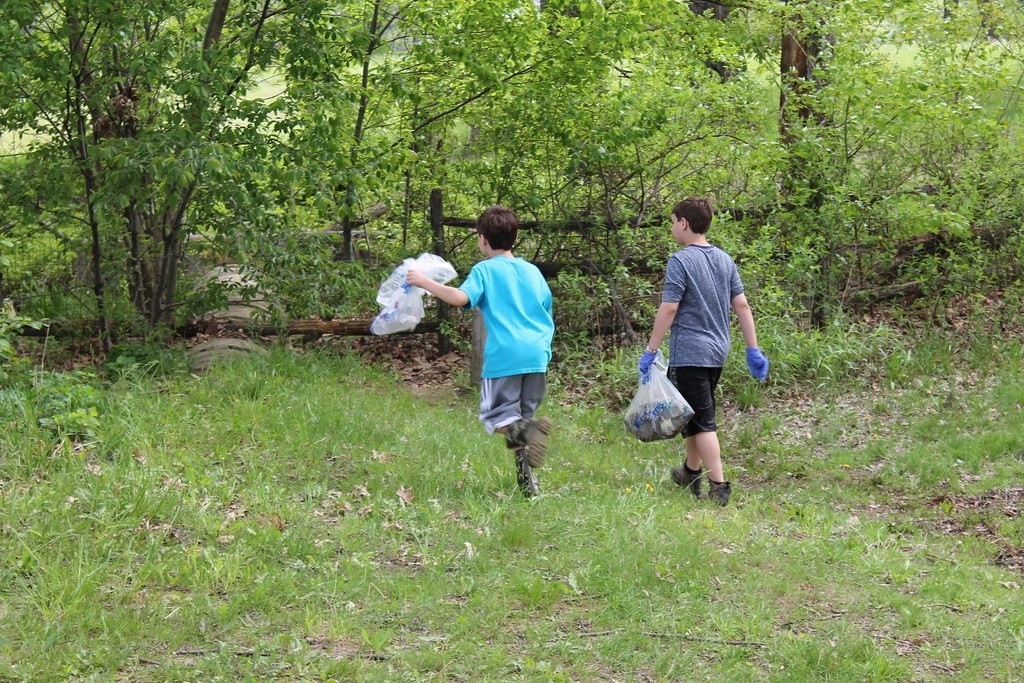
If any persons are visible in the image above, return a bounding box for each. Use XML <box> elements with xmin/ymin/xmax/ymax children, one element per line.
<box><xmin>638</xmin><ymin>196</ymin><xmax>770</xmax><ymax>509</ymax></box>
<box><xmin>406</xmin><ymin>205</ymin><xmax>555</xmax><ymax>499</ymax></box>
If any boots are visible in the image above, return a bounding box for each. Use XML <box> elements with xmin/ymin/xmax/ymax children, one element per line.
<box><xmin>505</xmin><ymin>417</ymin><xmax>553</xmax><ymax>468</ymax></box>
<box><xmin>697</xmin><ymin>479</ymin><xmax>731</xmax><ymax>508</ymax></box>
<box><xmin>672</xmin><ymin>458</ymin><xmax>702</xmax><ymax>491</ymax></box>
<box><xmin>514</xmin><ymin>448</ymin><xmax>538</xmax><ymax>499</ymax></box>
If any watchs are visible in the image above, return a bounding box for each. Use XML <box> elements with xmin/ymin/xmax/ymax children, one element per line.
<box><xmin>646</xmin><ymin>345</ymin><xmax>657</xmax><ymax>354</ymax></box>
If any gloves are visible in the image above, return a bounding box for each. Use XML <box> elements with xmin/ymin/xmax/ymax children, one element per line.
<box><xmin>639</xmin><ymin>346</ymin><xmax>657</xmax><ymax>375</ymax></box>
<box><xmin>746</xmin><ymin>348</ymin><xmax>769</xmax><ymax>381</ymax></box>
